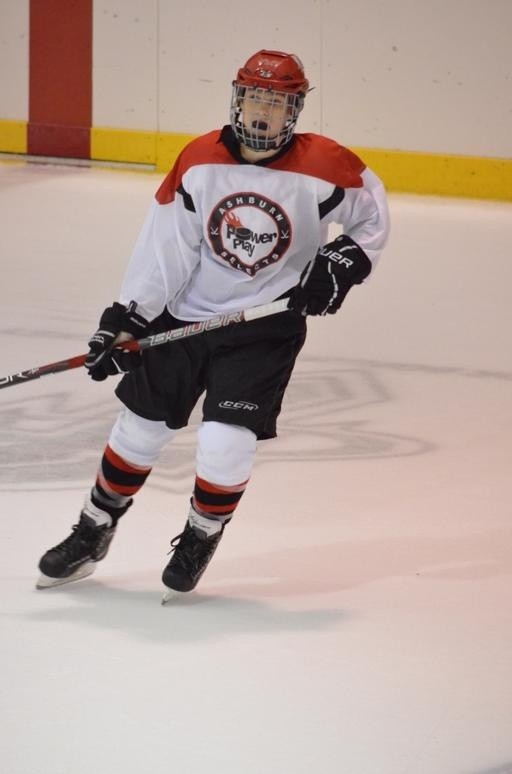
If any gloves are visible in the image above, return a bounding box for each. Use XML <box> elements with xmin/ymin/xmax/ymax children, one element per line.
<box><xmin>288</xmin><ymin>235</ymin><xmax>371</xmax><ymax>315</ymax></box>
<box><xmin>84</xmin><ymin>303</ymin><xmax>149</xmax><ymax>382</ymax></box>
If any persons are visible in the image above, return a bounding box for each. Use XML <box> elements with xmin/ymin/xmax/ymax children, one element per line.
<box><xmin>39</xmin><ymin>49</ymin><xmax>392</xmax><ymax>592</ymax></box>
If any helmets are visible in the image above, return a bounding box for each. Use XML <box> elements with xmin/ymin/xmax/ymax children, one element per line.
<box><xmin>229</xmin><ymin>49</ymin><xmax>309</xmax><ymax>151</ymax></box>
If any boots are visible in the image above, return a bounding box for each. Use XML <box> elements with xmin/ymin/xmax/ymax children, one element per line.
<box><xmin>38</xmin><ymin>499</ymin><xmax>134</xmax><ymax>577</ymax></box>
<box><xmin>162</xmin><ymin>498</ymin><xmax>232</xmax><ymax>592</ymax></box>
